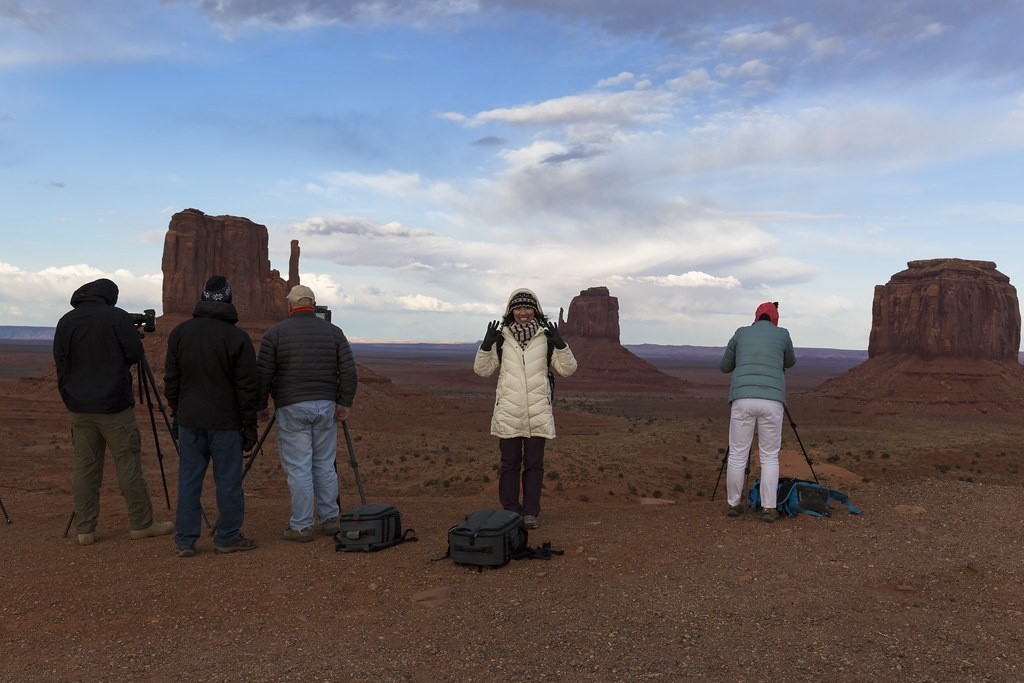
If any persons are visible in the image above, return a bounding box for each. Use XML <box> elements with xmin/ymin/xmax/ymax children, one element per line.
<box><xmin>473</xmin><ymin>288</ymin><xmax>577</xmax><ymax>529</ymax></box>
<box><xmin>52</xmin><ymin>279</ymin><xmax>174</xmax><ymax>545</ymax></box>
<box><xmin>719</xmin><ymin>302</ymin><xmax>797</xmax><ymax>523</ymax></box>
<box><xmin>257</xmin><ymin>285</ymin><xmax>357</xmax><ymax>542</ymax></box>
<box><xmin>164</xmin><ymin>276</ymin><xmax>257</xmax><ymax>557</ymax></box>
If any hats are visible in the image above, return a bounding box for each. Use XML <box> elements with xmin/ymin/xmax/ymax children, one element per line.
<box><xmin>201</xmin><ymin>276</ymin><xmax>232</xmax><ymax>305</ymax></box>
<box><xmin>287</xmin><ymin>284</ymin><xmax>315</xmax><ymax>306</ymax></box>
<box><xmin>755</xmin><ymin>301</ymin><xmax>779</xmax><ymax>326</ymax></box>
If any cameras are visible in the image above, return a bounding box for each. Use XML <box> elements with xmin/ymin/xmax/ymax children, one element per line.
<box><xmin>127</xmin><ymin>310</ymin><xmax>156</xmax><ymax>331</ymax></box>
<box><xmin>315</xmin><ymin>308</ymin><xmax>331</xmax><ymax>323</ymax></box>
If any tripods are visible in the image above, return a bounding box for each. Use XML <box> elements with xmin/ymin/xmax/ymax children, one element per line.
<box><xmin>63</xmin><ymin>332</ymin><xmax>211</xmax><ymax>537</ymax></box>
<box><xmin>712</xmin><ymin>405</ymin><xmax>819</xmax><ymax>501</ymax></box>
<box><xmin>209</xmin><ymin>409</ymin><xmax>367</xmax><ymax>536</ymax></box>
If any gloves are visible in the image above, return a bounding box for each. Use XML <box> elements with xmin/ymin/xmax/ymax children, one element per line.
<box><xmin>481</xmin><ymin>320</ymin><xmax>501</xmax><ymax>351</ymax></box>
<box><xmin>543</xmin><ymin>322</ymin><xmax>566</xmax><ymax>349</ymax></box>
<box><xmin>242</xmin><ymin>412</ymin><xmax>258</xmax><ymax>453</ymax></box>
<box><xmin>169</xmin><ymin>409</ymin><xmax>179</xmax><ymax>439</ymax></box>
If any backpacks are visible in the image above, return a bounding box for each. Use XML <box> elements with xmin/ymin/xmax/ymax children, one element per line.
<box><xmin>748</xmin><ymin>477</ymin><xmax>832</xmax><ymax>518</ymax></box>
<box><xmin>333</xmin><ymin>502</ymin><xmax>405</xmax><ymax>554</ymax></box>
<box><xmin>448</xmin><ymin>508</ymin><xmax>528</xmax><ymax>569</ymax></box>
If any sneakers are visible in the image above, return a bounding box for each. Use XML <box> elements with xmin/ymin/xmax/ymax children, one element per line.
<box><xmin>522</xmin><ymin>515</ymin><xmax>539</xmax><ymax>530</ymax></box>
<box><xmin>174</xmin><ymin>541</ymin><xmax>197</xmax><ymax>557</ymax></box>
<box><xmin>129</xmin><ymin>519</ymin><xmax>175</xmax><ymax>541</ymax></box>
<box><xmin>214</xmin><ymin>531</ymin><xmax>257</xmax><ymax>555</ymax></box>
<box><xmin>78</xmin><ymin>529</ymin><xmax>99</xmax><ymax>545</ymax></box>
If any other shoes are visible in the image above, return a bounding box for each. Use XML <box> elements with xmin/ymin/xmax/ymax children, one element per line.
<box><xmin>761</xmin><ymin>507</ymin><xmax>779</xmax><ymax>522</ymax></box>
<box><xmin>283</xmin><ymin>527</ymin><xmax>314</xmax><ymax>543</ymax></box>
<box><xmin>321</xmin><ymin>517</ymin><xmax>340</xmax><ymax>535</ymax></box>
<box><xmin>727</xmin><ymin>503</ymin><xmax>745</xmax><ymax>516</ymax></box>
